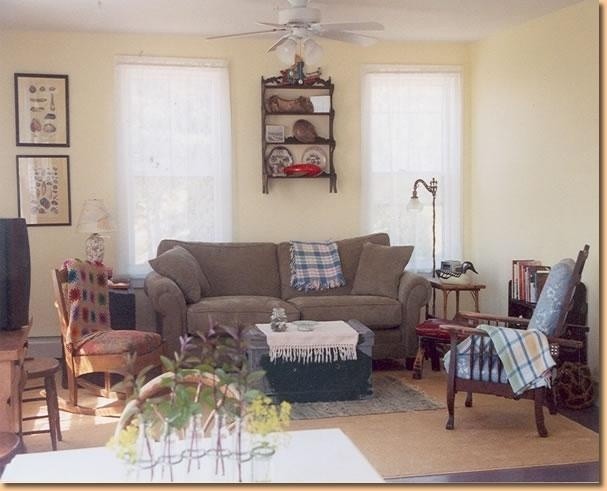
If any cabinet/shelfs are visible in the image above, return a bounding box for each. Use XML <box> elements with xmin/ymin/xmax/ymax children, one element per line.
<box><xmin>56</xmin><ymin>278</ymin><xmax>136</xmax><ymax>393</ymax></box>
<box><xmin>256</xmin><ymin>66</ymin><xmax>340</xmax><ymax>195</ymax></box>
<box><xmin>0</xmin><ymin>315</ymin><xmax>37</xmax><ymax>441</ymax></box>
<box><xmin>504</xmin><ymin>278</ymin><xmax>588</xmax><ymax>366</ymax></box>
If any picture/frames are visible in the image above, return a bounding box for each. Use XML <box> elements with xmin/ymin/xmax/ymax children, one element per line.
<box><xmin>13</xmin><ymin>154</ymin><xmax>74</xmax><ymax>230</ymax></box>
<box><xmin>11</xmin><ymin>70</ymin><xmax>71</xmax><ymax>149</ymax></box>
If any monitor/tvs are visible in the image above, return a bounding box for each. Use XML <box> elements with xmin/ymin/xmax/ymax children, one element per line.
<box><xmin>0</xmin><ymin>218</ymin><xmax>30</xmax><ymax>330</ymax></box>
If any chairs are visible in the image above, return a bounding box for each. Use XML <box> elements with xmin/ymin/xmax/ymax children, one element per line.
<box><xmin>413</xmin><ymin>243</ymin><xmax>591</xmax><ymax>439</ymax></box>
<box><xmin>40</xmin><ymin>256</ymin><xmax>169</xmax><ymax>426</ymax></box>
<box><xmin>109</xmin><ymin>366</ymin><xmax>255</xmax><ymax>446</ymax></box>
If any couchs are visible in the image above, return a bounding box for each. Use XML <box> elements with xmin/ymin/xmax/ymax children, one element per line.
<box><xmin>146</xmin><ymin>231</ymin><xmax>433</xmax><ymax>373</ymax></box>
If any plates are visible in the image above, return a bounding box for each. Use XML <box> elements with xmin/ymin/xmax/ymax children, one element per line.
<box><xmin>267</xmin><ymin>146</ymin><xmax>293</xmax><ymax>173</ymax></box>
<box><xmin>292</xmin><ymin>119</ymin><xmax>317</xmax><ymax>144</ymax></box>
<box><xmin>301</xmin><ymin>146</ymin><xmax>328</xmax><ymax>170</ymax></box>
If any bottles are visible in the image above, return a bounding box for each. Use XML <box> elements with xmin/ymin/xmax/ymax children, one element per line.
<box><xmin>270</xmin><ymin>307</ymin><xmax>288</xmax><ymax>333</ymax></box>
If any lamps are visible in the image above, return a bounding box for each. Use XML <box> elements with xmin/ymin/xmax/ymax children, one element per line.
<box><xmin>73</xmin><ymin>199</ymin><xmax>116</xmax><ymax>265</ymax></box>
<box><xmin>277</xmin><ymin>25</ymin><xmax>328</xmax><ymax>66</ymax></box>
<box><xmin>411</xmin><ymin>175</ymin><xmax>440</xmax><ymax>279</ymax></box>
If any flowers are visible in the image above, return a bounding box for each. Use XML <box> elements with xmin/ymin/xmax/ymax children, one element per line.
<box><xmin>105</xmin><ymin>312</ymin><xmax>298</xmax><ymax>486</ymax></box>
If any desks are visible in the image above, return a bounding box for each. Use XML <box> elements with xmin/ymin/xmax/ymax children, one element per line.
<box><xmin>239</xmin><ymin>319</ymin><xmax>376</xmax><ymax>403</ymax></box>
<box><xmin>422</xmin><ymin>275</ymin><xmax>488</xmax><ymax>328</ymax></box>
<box><xmin>0</xmin><ymin>425</ymin><xmax>391</xmax><ymax>487</ymax></box>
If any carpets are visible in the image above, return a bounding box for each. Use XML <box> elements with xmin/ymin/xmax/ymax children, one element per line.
<box><xmin>25</xmin><ymin>350</ymin><xmax>599</xmax><ymax>482</ymax></box>
<box><xmin>204</xmin><ymin>359</ymin><xmax>445</xmax><ymax>427</ymax></box>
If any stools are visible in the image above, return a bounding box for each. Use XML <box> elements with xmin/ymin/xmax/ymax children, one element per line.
<box><xmin>0</xmin><ymin>428</ymin><xmax>25</xmax><ymax>471</ymax></box>
<box><xmin>410</xmin><ymin>315</ymin><xmax>470</xmax><ymax>380</ymax></box>
<box><xmin>18</xmin><ymin>355</ymin><xmax>65</xmax><ymax>453</ymax></box>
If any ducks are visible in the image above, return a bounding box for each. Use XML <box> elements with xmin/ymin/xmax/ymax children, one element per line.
<box><xmin>435</xmin><ymin>260</ymin><xmax>480</xmax><ymax>285</ymax></box>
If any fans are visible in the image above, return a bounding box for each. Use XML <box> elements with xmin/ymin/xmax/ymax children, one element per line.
<box><xmin>205</xmin><ymin>0</ymin><xmax>387</xmax><ymax>57</ymax></box>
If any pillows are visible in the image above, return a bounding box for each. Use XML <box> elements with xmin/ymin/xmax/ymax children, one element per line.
<box><xmin>148</xmin><ymin>244</ymin><xmax>211</xmax><ymax>304</ymax></box>
<box><xmin>349</xmin><ymin>239</ymin><xmax>414</xmax><ymax>301</ymax></box>
